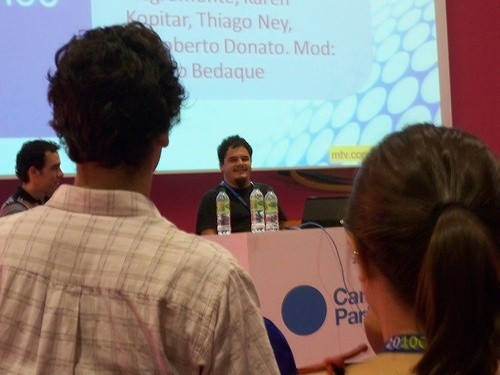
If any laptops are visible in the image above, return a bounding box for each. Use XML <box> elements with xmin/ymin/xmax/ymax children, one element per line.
<box><xmin>300</xmin><ymin>195</ymin><xmax>350</xmax><ymax>229</ymax></box>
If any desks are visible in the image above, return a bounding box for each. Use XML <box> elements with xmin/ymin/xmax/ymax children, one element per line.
<box><xmin>200</xmin><ymin>227</ymin><xmax>378</xmax><ymax>368</ymax></box>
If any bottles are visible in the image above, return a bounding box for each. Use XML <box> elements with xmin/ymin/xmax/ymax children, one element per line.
<box><xmin>216</xmin><ymin>188</ymin><xmax>231</xmax><ymax>235</ymax></box>
<box><xmin>264</xmin><ymin>187</ymin><xmax>279</xmax><ymax>232</ymax></box>
<box><xmin>250</xmin><ymin>183</ymin><xmax>265</xmax><ymax>233</ymax></box>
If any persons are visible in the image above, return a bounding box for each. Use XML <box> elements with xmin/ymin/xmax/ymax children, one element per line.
<box><xmin>0</xmin><ymin>139</ymin><xmax>65</xmax><ymax>218</ymax></box>
<box><xmin>329</xmin><ymin>123</ymin><xmax>500</xmax><ymax>375</ymax></box>
<box><xmin>196</xmin><ymin>134</ymin><xmax>287</xmax><ymax>236</ymax></box>
<box><xmin>0</xmin><ymin>20</ymin><xmax>283</xmax><ymax>375</ymax></box>
